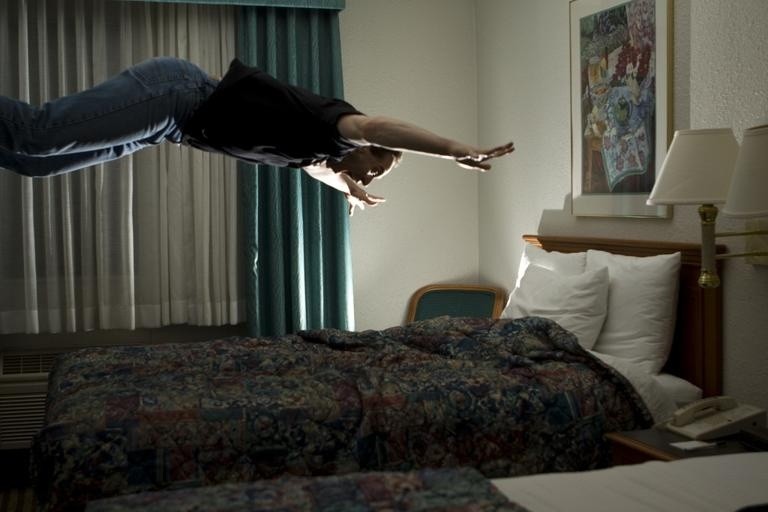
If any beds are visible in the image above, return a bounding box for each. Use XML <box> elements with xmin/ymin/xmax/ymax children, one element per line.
<box><xmin>43</xmin><ymin>233</ymin><xmax>727</xmax><ymax>511</ymax></box>
<box><xmin>85</xmin><ymin>450</ymin><xmax>768</xmax><ymax>511</ymax></box>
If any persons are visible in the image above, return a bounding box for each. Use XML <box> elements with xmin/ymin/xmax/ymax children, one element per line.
<box><xmin>1</xmin><ymin>55</ymin><xmax>518</xmax><ymax>218</ymax></box>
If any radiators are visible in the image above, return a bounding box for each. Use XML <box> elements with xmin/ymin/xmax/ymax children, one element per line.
<box><xmin>1</xmin><ymin>348</ymin><xmax>76</xmax><ymax>450</ymax></box>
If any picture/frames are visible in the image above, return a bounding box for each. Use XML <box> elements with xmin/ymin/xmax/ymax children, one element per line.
<box><xmin>570</xmin><ymin>0</ymin><xmax>677</xmax><ymax>223</ymax></box>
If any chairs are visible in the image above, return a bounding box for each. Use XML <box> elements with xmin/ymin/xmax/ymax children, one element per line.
<box><xmin>405</xmin><ymin>281</ymin><xmax>504</xmax><ymax>325</ymax></box>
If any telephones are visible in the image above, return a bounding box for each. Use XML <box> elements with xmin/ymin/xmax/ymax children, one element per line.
<box><xmin>664</xmin><ymin>395</ymin><xmax>767</xmax><ymax>441</ymax></box>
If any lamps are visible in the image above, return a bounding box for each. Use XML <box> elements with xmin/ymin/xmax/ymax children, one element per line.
<box><xmin>643</xmin><ymin>123</ymin><xmax>768</xmax><ymax>287</ymax></box>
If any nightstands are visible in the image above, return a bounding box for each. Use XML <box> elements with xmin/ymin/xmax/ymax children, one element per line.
<box><xmin>602</xmin><ymin>427</ymin><xmax>768</xmax><ymax>467</ymax></box>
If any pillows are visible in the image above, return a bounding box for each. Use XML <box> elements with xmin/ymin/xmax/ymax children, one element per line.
<box><xmin>516</xmin><ymin>239</ymin><xmax>585</xmax><ymax>287</ymax></box>
<box><xmin>585</xmin><ymin>248</ymin><xmax>680</xmax><ymax>378</ymax></box>
<box><xmin>498</xmin><ymin>262</ymin><xmax>612</xmax><ymax>350</ymax></box>
